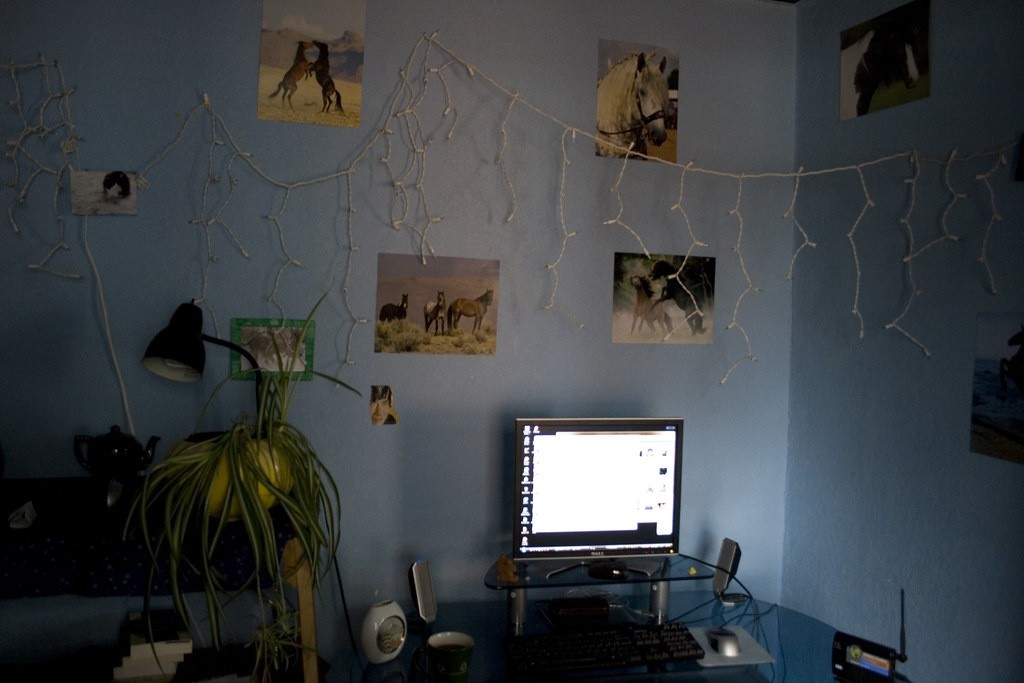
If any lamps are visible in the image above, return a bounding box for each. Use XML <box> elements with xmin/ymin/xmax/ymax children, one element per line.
<box><xmin>142</xmin><ymin>297</ymin><xmax>264</xmax><ymax>418</ymax></box>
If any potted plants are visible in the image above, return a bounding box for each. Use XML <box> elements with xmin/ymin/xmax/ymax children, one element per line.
<box><xmin>122</xmin><ymin>287</ymin><xmax>363</xmax><ymax>683</ymax></box>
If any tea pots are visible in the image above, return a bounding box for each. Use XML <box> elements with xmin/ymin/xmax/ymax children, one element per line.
<box><xmin>73</xmin><ymin>425</ymin><xmax>161</xmax><ymax>478</ymax></box>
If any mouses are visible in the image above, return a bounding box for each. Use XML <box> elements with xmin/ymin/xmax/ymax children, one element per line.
<box><xmin>707</xmin><ymin>628</ymin><xmax>741</xmax><ymax>657</ymax></box>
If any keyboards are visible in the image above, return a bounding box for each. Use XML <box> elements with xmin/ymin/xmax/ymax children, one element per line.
<box><xmin>499</xmin><ymin>622</ymin><xmax>706</xmax><ymax>683</ymax></box>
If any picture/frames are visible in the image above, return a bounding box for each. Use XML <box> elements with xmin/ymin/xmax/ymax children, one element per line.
<box><xmin>229</xmin><ymin>317</ymin><xmax>315</xmax><ymax>382</ymax></box>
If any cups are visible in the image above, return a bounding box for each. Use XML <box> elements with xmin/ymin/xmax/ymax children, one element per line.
<box><xmin>410</xmin><ymin>631</ymin><xmax>475</xmax><ymax>683</ymax></box>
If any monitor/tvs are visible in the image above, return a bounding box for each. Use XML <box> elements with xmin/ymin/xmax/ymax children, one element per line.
<box><xmin>514</xmin><ymin>418</ymin><xmax>685</xmax><ymax>581</ymax></box>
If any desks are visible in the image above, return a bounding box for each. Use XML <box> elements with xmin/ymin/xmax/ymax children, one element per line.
<box><xmin>0</xmin><ymin>476</ymin><xmax>316</xmax><ymax>683</ymax></box>
<box><xmin>358</xmin><ymin>593</ymin><xmax>913</xmax><ymax>683</ymax></box>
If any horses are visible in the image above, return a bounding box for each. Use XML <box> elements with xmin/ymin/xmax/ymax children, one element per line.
<box><xmin>647</xmin><ymin>260</ymin><xmax>706</xmax><ymax>336</ymax></box>
<box><xmin>309</xmin><ymin>40</ymin><xmax>344</xmax><ymax>113</ymax></box>
<box><xmin>378</xmin><ymin>293</ymin><xmax>409</xmax><ymax>325</ymax></box>
<box><xmin>268</xmin><ymin>40</ymin><xmax>313</xmax><ymax>110</ymax></box>
<box><xmin>447</xmin><ymin>289</ymin><xmax>495</xmax><ymax>333</ymax></box>
<box><xmin>840</xmin><ymin>21</ymin><xmax>918</xmax><ymax>119</ymax></box>
<box><xmin>424</xmin><ymin>289</ymin><xmax>445</xmax><ymax>335</ymax></box>
<box><xmin>630</xmin><ymin>276</ymin><xmax>674</xmax><ymax>337</ymax></box>
<box><xmin>596</xmin><ymin>53</ymin><xmax>669</xmax><ymax>161</ymax></box>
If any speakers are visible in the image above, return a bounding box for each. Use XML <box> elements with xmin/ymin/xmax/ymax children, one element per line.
<box><xmin>409</xmin><ymin>559</ymin><xmax>437</xmax><ymax>646</ymax></box>
<box><xmin>712</xmin><ymin>538</ymin><xmax>746</xmax><ymax>605</ymax></box>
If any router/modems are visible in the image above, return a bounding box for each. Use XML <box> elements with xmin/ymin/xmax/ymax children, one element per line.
<box><xmin>831</xmin><ymin>588</ymin><xmax>907</xmax><ymax>683</ymax></box>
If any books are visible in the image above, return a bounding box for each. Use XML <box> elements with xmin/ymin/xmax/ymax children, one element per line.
<box><xmin>111</xmin><ymin>673</ymin><xmax>174</xmax><ymax>683</ymax></box>
<box><xmin>103</xmin><ymin>609</ymin><xmax>192</xmax><ymax>679</ymax></box>
